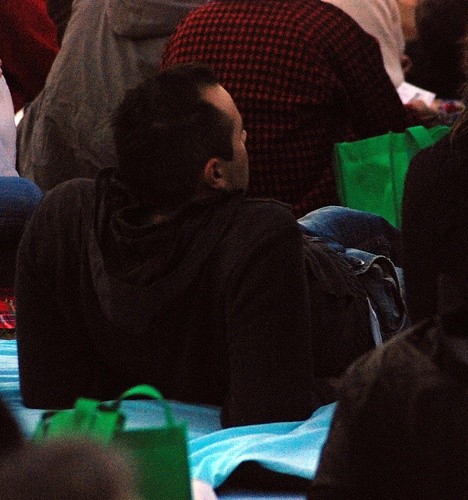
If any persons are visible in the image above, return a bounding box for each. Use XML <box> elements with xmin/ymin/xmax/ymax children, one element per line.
<box><xmin>0</xmin><ymin>0</ymin><xmax>463</xmax><ymax>299</ymax></box>
<box><xmin>13</xmin><ymin>63</ymin><xmax>409</xmax><ymax>429</ymax></box>
<box><xmin>305</xmin><ymin>0</ymin><xmax>468</xmax><ymax>500</ymax></box>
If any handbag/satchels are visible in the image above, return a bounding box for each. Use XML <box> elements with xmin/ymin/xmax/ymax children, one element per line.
<box><xmin>32</xmin><ymin>385</ymin><xmax>193</xmax><ymax>500</ymax></box>
<box><xmin>333</xmin><ymin>125</ymin><xmax>450</xmax><ymax>231</ymax></box>
